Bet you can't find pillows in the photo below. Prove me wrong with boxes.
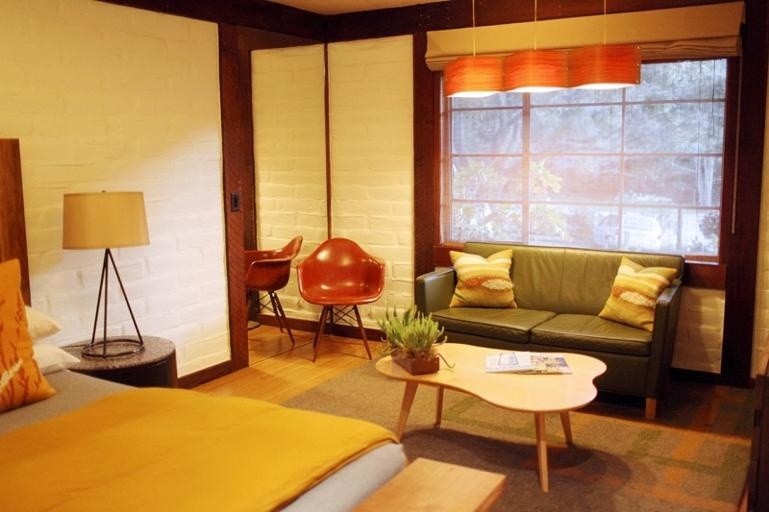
[448,248,517,310]
[24,306,60,341]
[31,342,80,375]
[601,254,676,333]
[0,257,55,414]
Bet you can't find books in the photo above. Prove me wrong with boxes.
[487,349,570,375]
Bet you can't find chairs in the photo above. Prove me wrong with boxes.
[244,235,302,344]
[298,238,384,361]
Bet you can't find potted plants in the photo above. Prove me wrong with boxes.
[377,305,456,374]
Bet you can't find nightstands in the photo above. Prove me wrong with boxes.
[55,335,177,388]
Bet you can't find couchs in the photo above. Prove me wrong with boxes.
[415,241,685,421]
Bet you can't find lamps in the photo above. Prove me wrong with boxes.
[443,56,501,100]
[504,48,567,95]
[570,45,642,92]
[57,189,149,359]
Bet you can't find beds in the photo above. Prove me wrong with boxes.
[0,138,407,512]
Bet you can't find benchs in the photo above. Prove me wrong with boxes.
[353,455,509,512]
